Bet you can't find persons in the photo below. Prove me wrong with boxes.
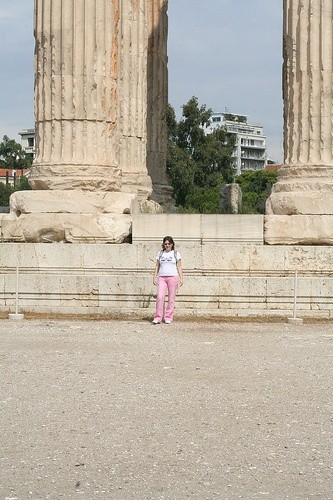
[152,236,184,325]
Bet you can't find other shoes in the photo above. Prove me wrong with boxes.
[164,320,171,324]
[151,318,162,324]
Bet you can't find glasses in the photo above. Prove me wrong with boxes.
[163,243,172,245]
